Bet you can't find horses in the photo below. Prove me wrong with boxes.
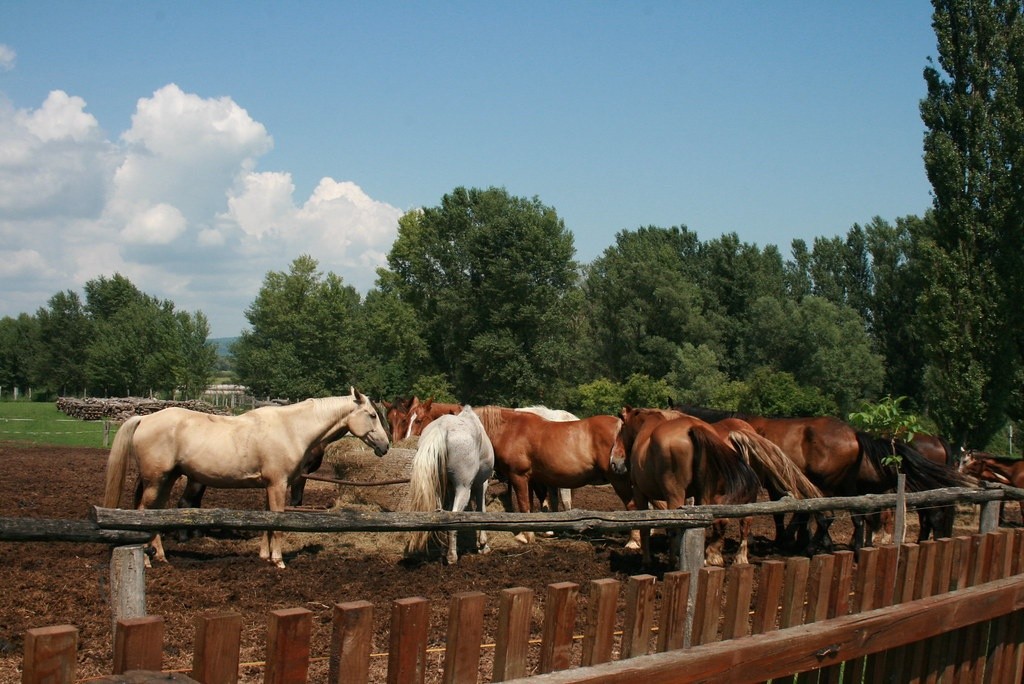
[472,406,643,550]
[609,406,765,568]
[404,404,494,566]
[380,395,413,447]
[513,404,581,536]
[402,395,463,439]
[739,415,988,563]
[958,445,1024,525]
[644,407,832,565]
[103,384,389,570]
[844,425,957,564]
